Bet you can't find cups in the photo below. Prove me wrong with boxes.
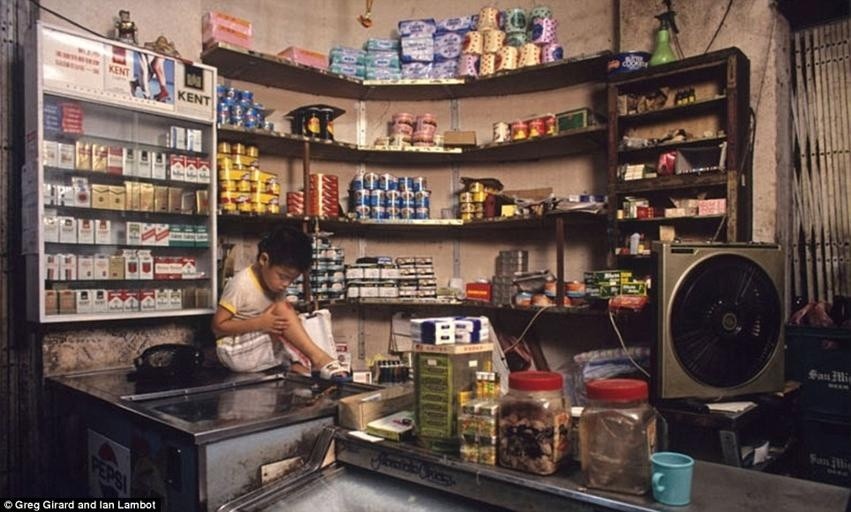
[648,451,696,507]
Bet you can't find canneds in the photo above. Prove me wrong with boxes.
[352,172,431,218]
[218,141,280,215]
[457,181,486,218]
[514,281,584,307]
[373,113,439,148]
[284,234,347,299]
[216,84,266,129]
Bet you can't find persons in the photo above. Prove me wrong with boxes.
[130,51,170,103]
[114,9,138,45]
[211,229,352,383]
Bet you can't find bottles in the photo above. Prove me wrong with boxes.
[498,370,573,478]
[578,378,657,497]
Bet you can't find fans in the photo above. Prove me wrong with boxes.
[657,243,787,399]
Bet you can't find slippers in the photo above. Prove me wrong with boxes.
[310,359,353,382]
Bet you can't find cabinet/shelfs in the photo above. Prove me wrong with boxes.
[24,20,218,324]
[465,50,660,322]
[201,42,363,313]
[608,46,750,262]
[364,76,465,307]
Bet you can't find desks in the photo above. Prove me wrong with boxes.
[610,371,813,479]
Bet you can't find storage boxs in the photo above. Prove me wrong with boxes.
[787,326,851,420]
[795,411,851,488]
